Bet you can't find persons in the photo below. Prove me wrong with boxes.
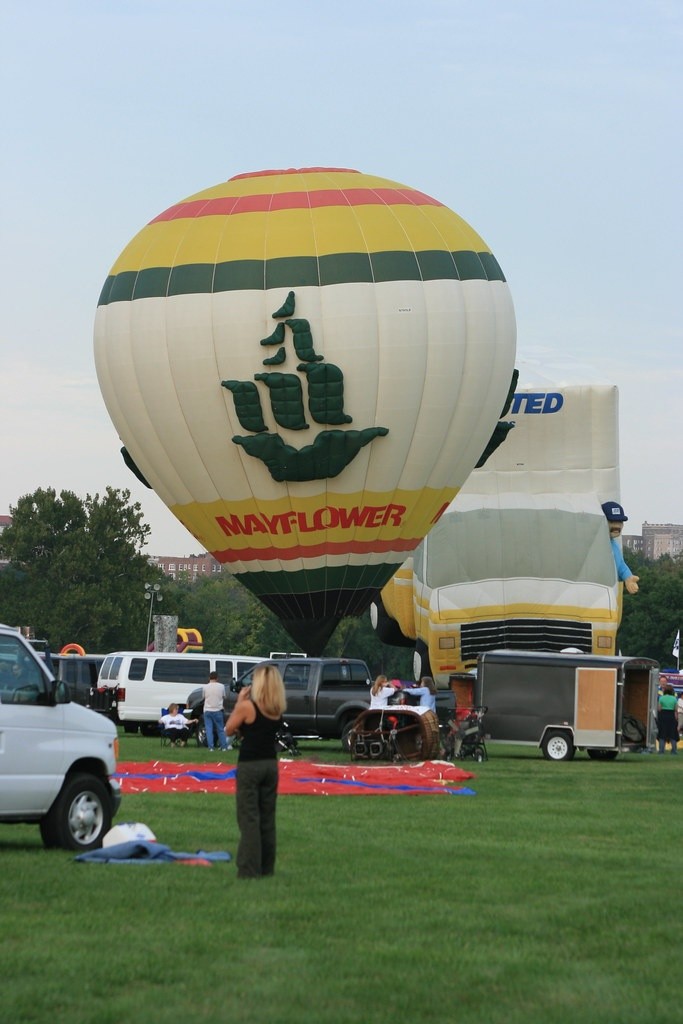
[226,665,287,880]
[601,502,639,594]
[657,675,683,755]
[186,672,228,752]
[402,677,437,713]
[388,679,405,705]
[158,703,199,747]
[370,675,397,708]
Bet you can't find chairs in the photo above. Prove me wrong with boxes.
[443,705,488,763]
[160,708,184,747]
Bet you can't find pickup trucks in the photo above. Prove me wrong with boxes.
[195,657,456,752]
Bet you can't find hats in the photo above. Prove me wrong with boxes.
[602,502,628,521]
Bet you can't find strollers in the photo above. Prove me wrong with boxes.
[441,706,487,761]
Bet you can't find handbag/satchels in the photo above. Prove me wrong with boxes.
[622,714,646,741]
[103,823,158,850]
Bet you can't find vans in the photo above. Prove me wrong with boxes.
[89,649,304,736]
[0,624,119,850]
[0,652,108,701]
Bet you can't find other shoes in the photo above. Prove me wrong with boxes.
[657,751,664,754]
[671,750,678,754]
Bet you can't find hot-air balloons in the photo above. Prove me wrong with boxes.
[95,167,516,661]
